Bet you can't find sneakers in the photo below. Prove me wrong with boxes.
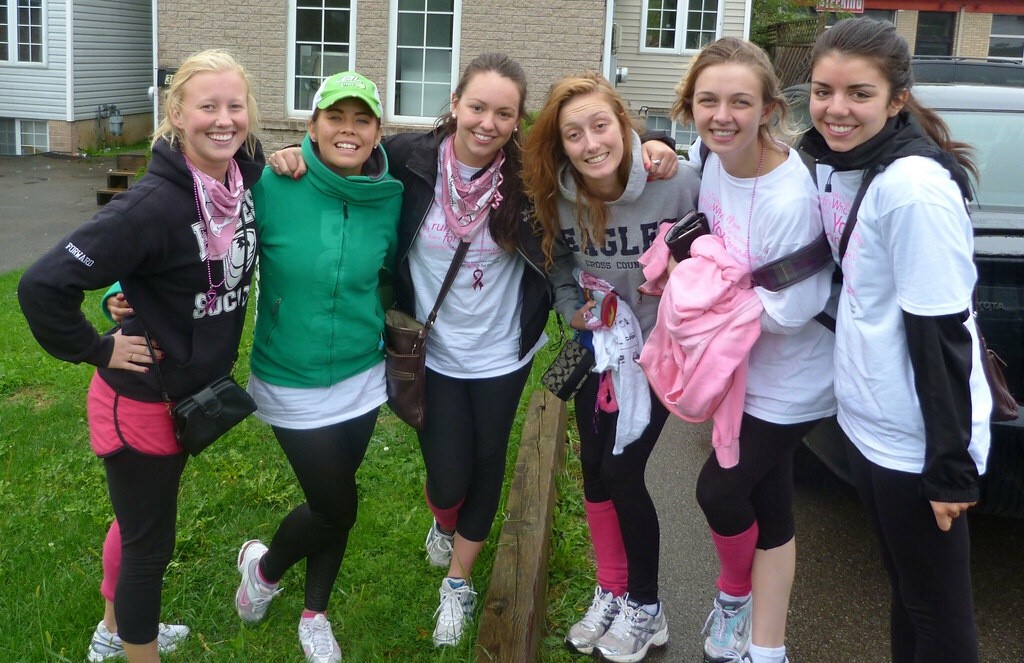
[235,539,279,622]
[299,610,343,663]
[565,587,669,663]
[431,578,477,647]
[87,618,191,663]
[702,591,789,663]
[427,519,454,568]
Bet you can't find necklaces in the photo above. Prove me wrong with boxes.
[442,139,502,289]
[718,148,766,282]
[182,152,234,313]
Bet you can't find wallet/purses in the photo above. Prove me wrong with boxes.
[540,339,597,402]
[664,210,711,263]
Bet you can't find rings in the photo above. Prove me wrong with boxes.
[273,164,279,167]
[651,159,661,165]
[129,352,133,361]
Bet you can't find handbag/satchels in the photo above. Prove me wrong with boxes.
[385,308,430,431]
[979,336,1018,422]
[170,376,259,457]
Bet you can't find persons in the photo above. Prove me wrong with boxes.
[669,36,844,663]
[800,17,994,663]
[18,49,265,663]
[102,72,405,663]
[520,70,703,663]
[268,52,679,645]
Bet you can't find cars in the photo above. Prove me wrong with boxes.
[910,57,1023,431]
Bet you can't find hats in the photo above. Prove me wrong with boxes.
[312,72,383,119]
